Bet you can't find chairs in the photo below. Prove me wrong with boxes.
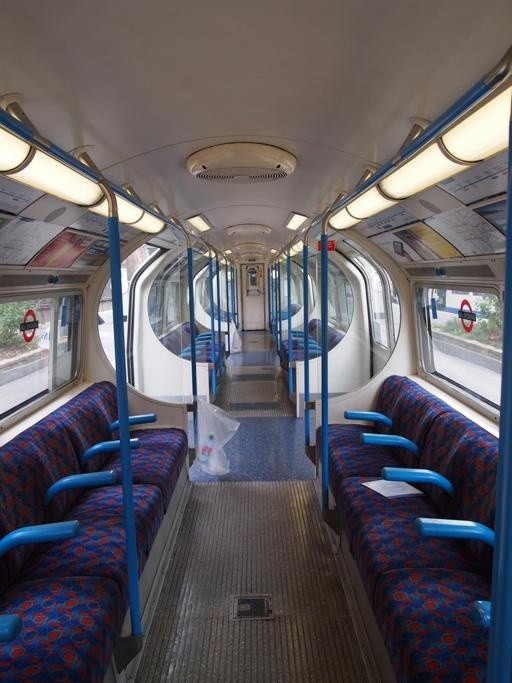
[268,304,303,338]
[279,318,345,406]
[315,374,501,680]
[1,380,188,683]
[157,319,230,402]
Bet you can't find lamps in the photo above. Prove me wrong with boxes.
[328,84,512,231]
[0,126,165,233]
